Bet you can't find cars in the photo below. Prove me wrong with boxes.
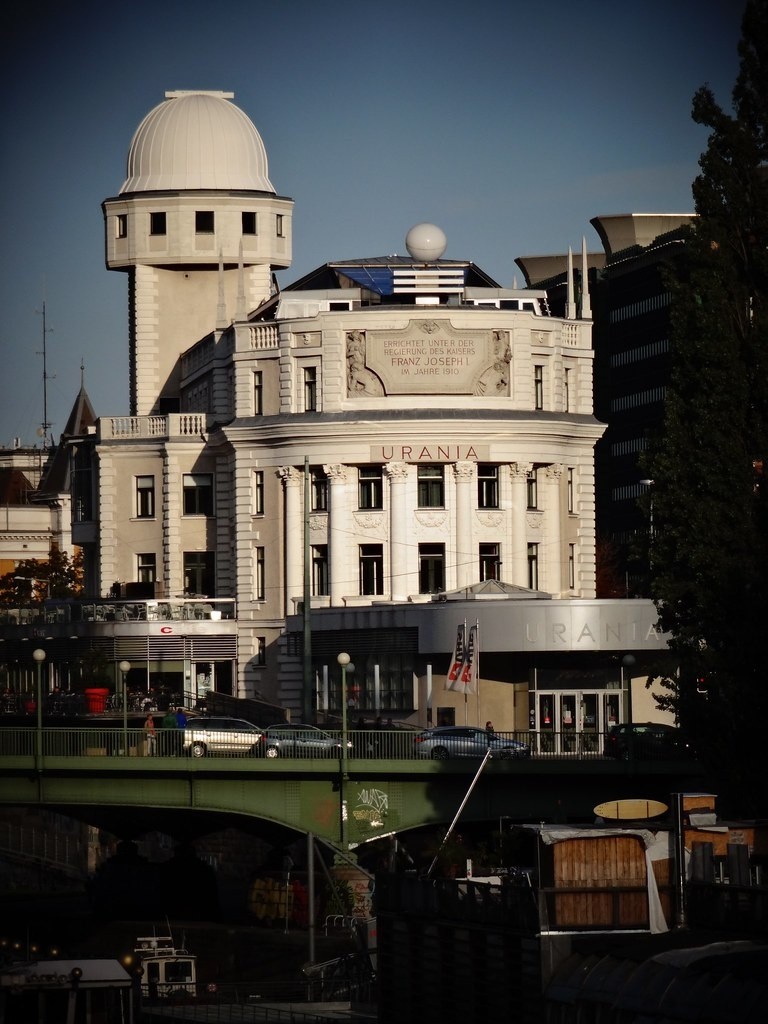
[255,722,355,760]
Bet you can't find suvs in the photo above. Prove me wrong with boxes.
[603,722,693,764]
[178,717,264,758]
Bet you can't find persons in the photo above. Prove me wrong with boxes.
[486,721,494,733]
[143,714,158,757]
[163,708,187,728]
[356,717,395,731]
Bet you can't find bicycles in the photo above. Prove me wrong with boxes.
[0,690,156,713]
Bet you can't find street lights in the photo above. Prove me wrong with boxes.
[118,661,131,757]
[333,651,360,867]
[33,648,46,802]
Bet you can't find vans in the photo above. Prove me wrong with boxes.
[411,725,532,762]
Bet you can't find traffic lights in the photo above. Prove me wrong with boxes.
[696,674,709,693]
